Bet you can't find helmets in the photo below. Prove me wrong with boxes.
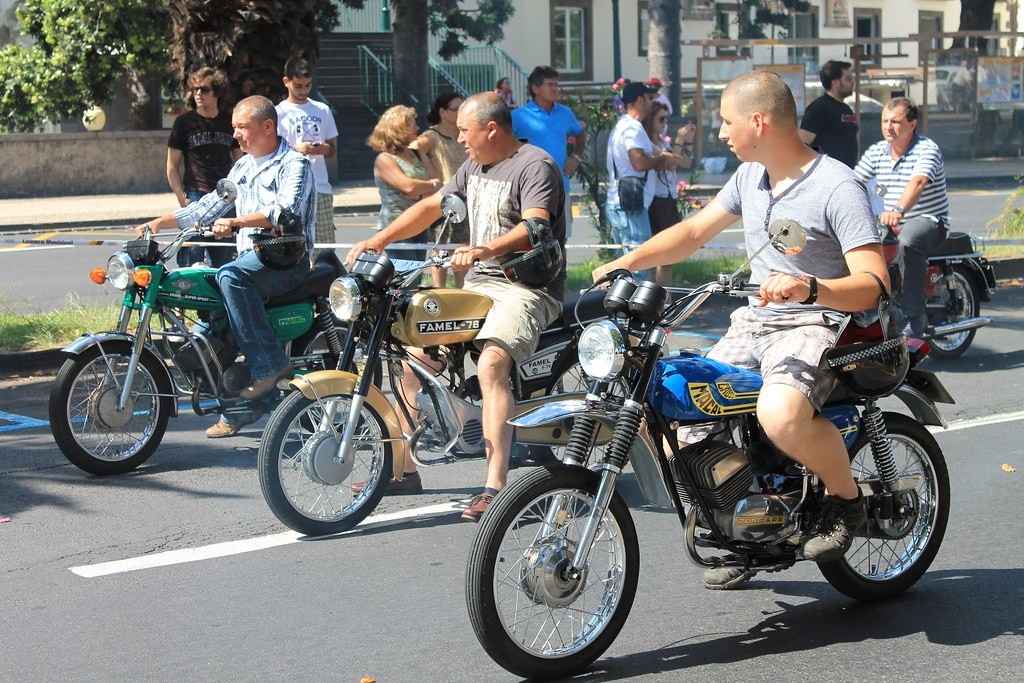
[248,209,306,270]
[501,239,563,289]
[825,293,910,398]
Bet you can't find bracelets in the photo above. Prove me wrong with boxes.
[431,179,438,193]
[684,143,694,145]
[675,143,683,148]
[570,153,582,164]
[799,275,818,305]
[683,147,695,160]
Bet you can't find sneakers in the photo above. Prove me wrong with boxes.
[239,360,292,400]
[461,493,497,521]
[206,411,264,437]
[352,471,422,496]
[803,485,867,562]
[704,566,792,590]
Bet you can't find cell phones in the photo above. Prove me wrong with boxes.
[312,142,320,146]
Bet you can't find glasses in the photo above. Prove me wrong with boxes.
[654,117,665,123]
[191,86,213,95]
[448,108,458,112]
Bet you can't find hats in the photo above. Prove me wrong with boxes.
[621,82,657,102]
[612,79,624,90]
[646,78,661,86]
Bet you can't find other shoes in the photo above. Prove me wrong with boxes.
[902,315,929,337]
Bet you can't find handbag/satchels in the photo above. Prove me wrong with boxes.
[618,176,644,211]
[647,193,679,236]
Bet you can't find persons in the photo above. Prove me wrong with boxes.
[167,68,244,333]
[606,77,696,318]
[494,77,518,111]
[365,105,440,291]
[952,60,975,109]
[273,59,339,267]
[591,68,891,590]
[410,92,471,290]
[135,95,317,439]
[798,60,859,169]
[511,66,585,245]
[346,91,567,522]
[854,96,950,336]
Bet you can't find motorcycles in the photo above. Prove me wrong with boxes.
[48,178,384,477]
[870,184,996,360]
[257,193,670,536]
[464,217,994,683]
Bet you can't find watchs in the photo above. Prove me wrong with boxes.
[892,206,906,218]
